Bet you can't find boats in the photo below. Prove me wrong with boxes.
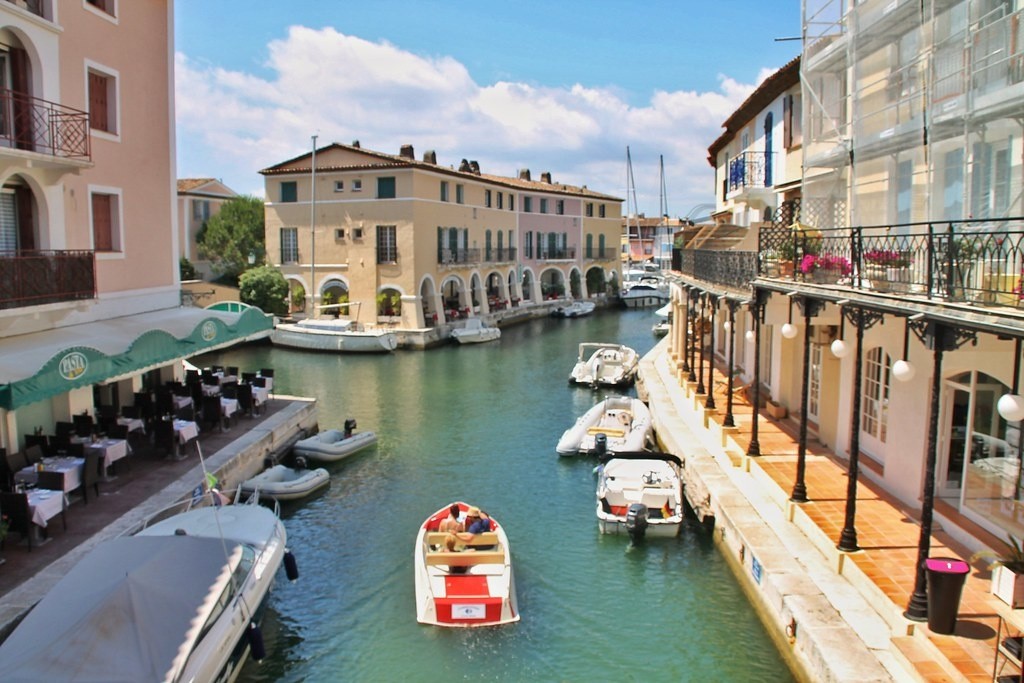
[652,320,670,337]
[568,342,641,388]
[594,453,685,543]
[292,418,379,462]
[555,394,653,456]
[0,483,300,682]
[554,301,595,317]
[239,465,329,502]
[414,501,519,628]
[450,319,501,345]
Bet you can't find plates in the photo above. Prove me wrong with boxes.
[42,456,76,469]
[26,488,51,506]
[70,437,115,448]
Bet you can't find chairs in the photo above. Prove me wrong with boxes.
[715,365,754,406]
[0,365,276,554]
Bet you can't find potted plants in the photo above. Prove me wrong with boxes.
[766,400,786,419]
[968,533,1024,609]
[756,238,798,279]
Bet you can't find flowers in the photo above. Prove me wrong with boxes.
[1011,267,1024,301]
[797,214,1008,278]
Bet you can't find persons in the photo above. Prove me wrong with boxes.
[438,504,495,575]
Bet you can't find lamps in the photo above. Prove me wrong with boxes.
[781,295,1023,422]
[695,290,756,343]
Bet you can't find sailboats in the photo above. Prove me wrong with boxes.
[617,145,671,307]
[269,134,398,353]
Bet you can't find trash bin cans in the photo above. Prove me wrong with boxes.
[921,556,971,636]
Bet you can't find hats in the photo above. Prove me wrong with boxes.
[466,506,479,517]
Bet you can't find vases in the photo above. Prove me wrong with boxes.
[812,264,913,295]
[940,262,970,302]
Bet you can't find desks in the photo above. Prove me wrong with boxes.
[16,488,70,543]
[14,455,88,505]
[115,417,146,444]
[250,386,270,418]
[200,383,221,397]
[220,397,242,430]
[920,556,970,635]
[213,372,237,386]
[70,436,133,477]
[171,420,200,455]
[256,375,274,400]
[149,393,194,420]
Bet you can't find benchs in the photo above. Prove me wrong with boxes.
[426,533,505,576]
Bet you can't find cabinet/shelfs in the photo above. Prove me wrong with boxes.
[991,609,1024,683]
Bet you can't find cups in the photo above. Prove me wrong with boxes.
[33,463,38,473]
[92,434,96,442]
[38,464,43,472]
